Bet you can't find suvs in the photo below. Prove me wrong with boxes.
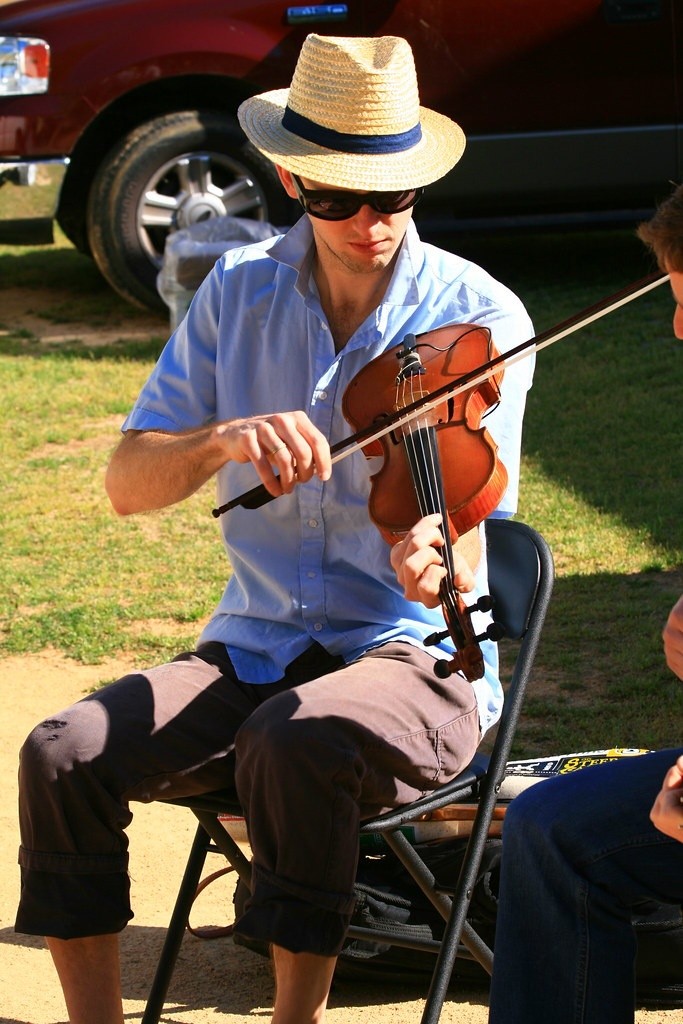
[0,0,683,320]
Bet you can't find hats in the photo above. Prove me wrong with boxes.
[236,32,467,193]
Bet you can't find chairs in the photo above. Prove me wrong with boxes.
[140,518,556,1024]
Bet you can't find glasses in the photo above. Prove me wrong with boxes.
[290,172,424,221]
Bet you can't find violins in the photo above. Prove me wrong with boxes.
[345,324,505,681]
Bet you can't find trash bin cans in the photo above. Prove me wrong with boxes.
[153,221,294,345]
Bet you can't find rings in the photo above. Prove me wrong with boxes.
[270,443,286,456]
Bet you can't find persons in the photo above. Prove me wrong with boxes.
[11,33,539,1024]
[488,185,683,1024]
